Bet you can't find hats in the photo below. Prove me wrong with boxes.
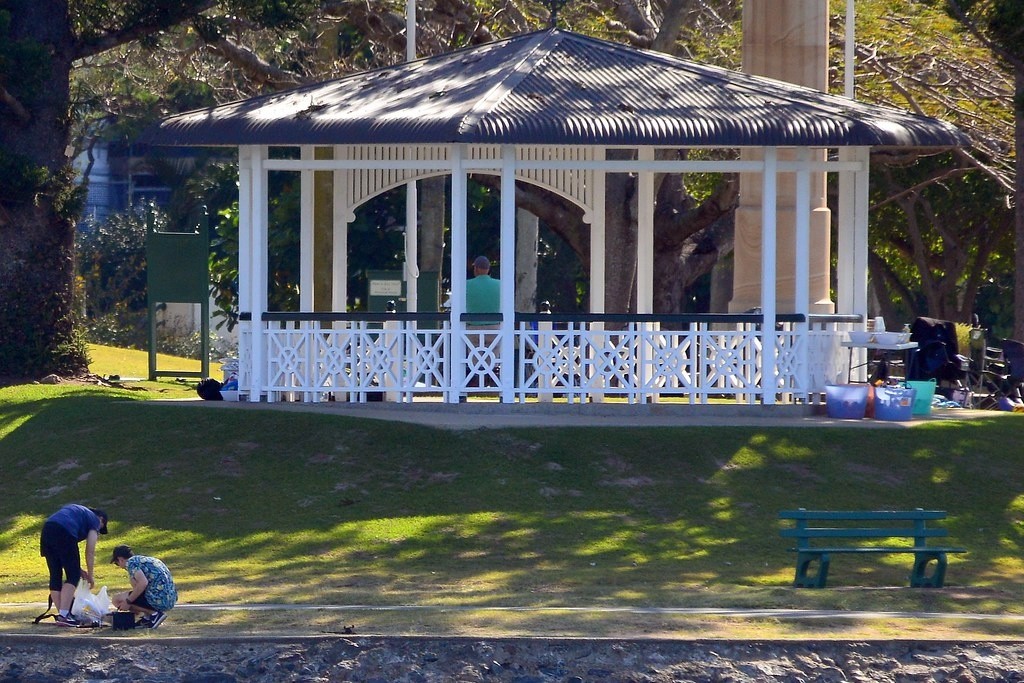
[94,508,108,534]
[471,256,490,269]
[109,545,132,564]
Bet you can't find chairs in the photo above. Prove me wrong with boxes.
[966,339,1024,410]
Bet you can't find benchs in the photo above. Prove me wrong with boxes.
[776,508,968,592]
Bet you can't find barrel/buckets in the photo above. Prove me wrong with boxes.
[822,377,871,419]
[874,383,916,421]
[897,377,936,415]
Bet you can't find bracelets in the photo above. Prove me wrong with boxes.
[126,599,135,604]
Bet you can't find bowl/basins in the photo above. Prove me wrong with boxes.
[873,332,912,345]
[848,331,874,343]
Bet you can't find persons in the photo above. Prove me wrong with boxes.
[466,255,500,387]
[197,373,288,403]
[442,283,451,313]
[112,544,178,629]
[531,301,557,348]
[40,502,108,626]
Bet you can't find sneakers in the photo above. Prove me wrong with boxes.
[56,612,79,626]
[134,616,152,628]
[147,609,166,628]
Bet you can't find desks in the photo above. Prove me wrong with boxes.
[840,340,919,385]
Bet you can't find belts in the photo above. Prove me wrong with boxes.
[466,322,500,326]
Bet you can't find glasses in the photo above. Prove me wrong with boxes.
[114,559,120,566]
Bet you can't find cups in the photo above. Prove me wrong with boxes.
[874,316,886,333]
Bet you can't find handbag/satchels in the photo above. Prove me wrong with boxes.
[70,577,110,627]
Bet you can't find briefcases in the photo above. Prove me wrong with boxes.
[111,607,136,630]
